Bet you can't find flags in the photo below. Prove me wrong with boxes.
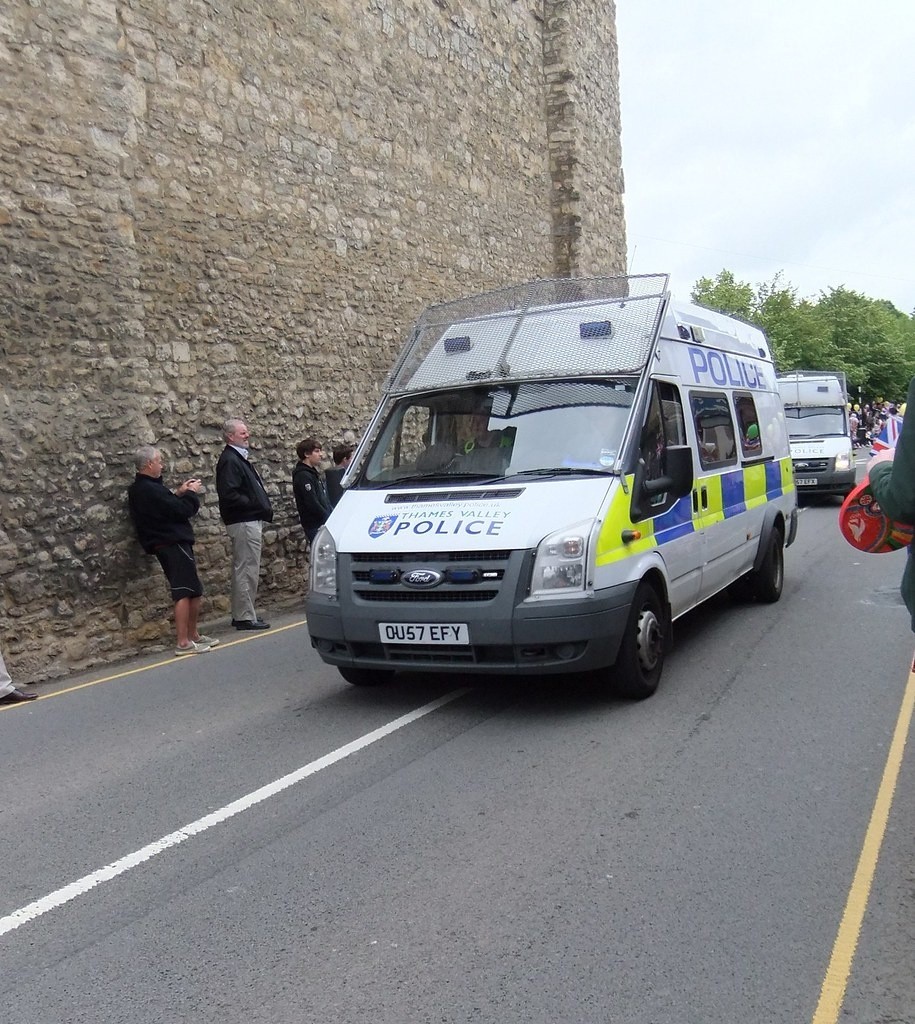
[870,417,904,457]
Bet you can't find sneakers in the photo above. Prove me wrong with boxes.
[195,635,219,647]
[174,640,210,655]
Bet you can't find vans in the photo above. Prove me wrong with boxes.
[775,369,867,503]
[299,272,801,700]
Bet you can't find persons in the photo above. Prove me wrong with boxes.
[458,412,514,456]
[216,420,273,629]
[849,400,902,449]
[0,652,38,703]
[325,444,352,507]
[866,376,915,673]
[291,438,335,544]
[127,446,219,656]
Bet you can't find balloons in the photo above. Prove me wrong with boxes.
[748,424,758,438]
[854,404,859,410]
[847,403,852,409]
[884,401,889,406]
[900,403,907,416]
[876,397,883,403]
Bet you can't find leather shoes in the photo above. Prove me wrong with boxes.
[232,617,263,627]
[236,619,270,630]
[0,688,38,705]
